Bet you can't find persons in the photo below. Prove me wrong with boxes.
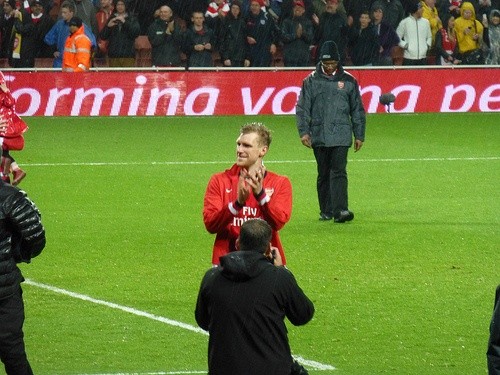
[0,0,500,72]
[202,123,293,269]
[0,71,29,186]
[296,40,366,223]
[195,218,315,374]
[0,181,46,375]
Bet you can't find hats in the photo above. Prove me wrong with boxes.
[30,0,42,7]
[293,0,304,7]
[319,41,340,60]
[67,16,82,27]
[253,0,264,6]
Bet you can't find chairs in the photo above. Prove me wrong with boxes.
[0,37,440,69]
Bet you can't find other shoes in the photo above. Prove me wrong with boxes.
[320,212,332,219]
[334,210,354,222]
